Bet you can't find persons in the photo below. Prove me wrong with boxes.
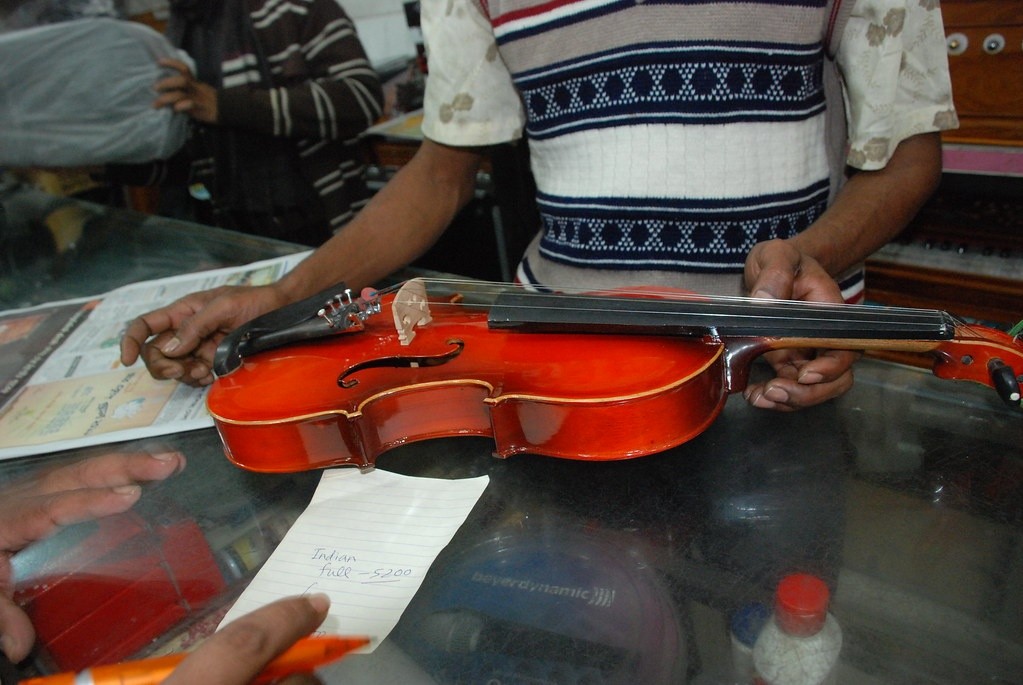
[0,449,333,685]
[113,1,960,418]
[100,0,387,252]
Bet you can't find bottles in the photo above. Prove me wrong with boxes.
[729,602,774,678]
[751,572,844,683]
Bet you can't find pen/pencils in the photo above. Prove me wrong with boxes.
[14,633,378,685]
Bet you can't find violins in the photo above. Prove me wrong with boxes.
[206,278,1023,473]
